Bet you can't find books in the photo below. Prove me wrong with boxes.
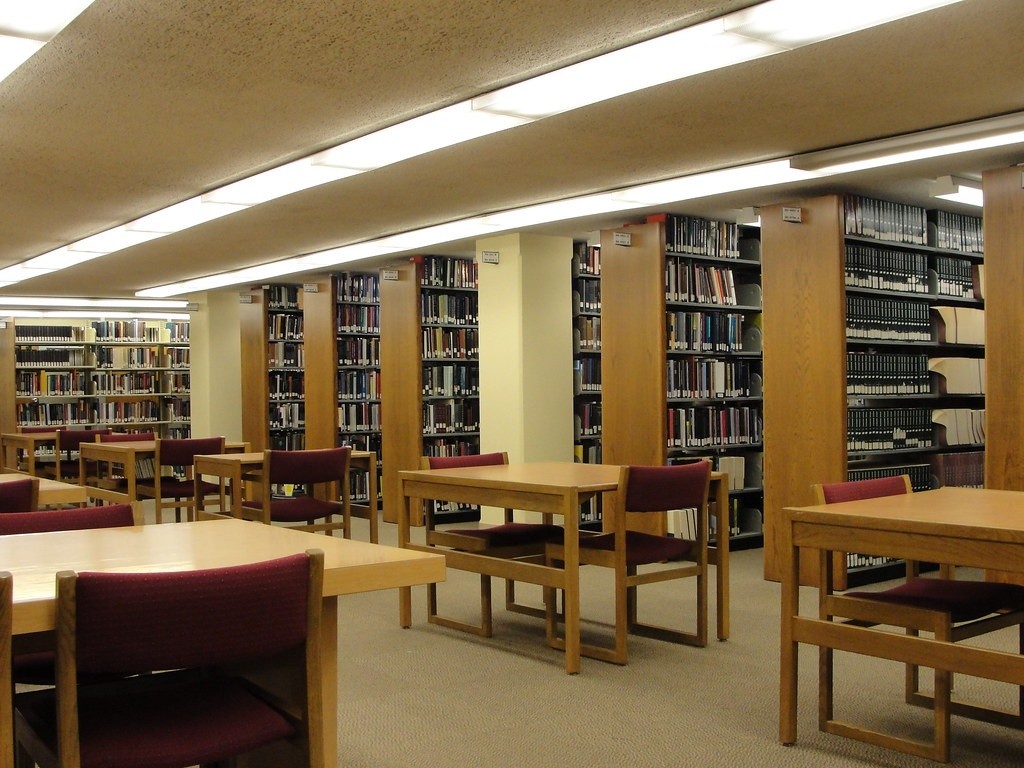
[6,192,988,578]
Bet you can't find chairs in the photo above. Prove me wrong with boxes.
[0,571,15,768]
[0,425,1024,767]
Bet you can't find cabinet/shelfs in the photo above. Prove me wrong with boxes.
[239,283,309,504]
[303,271,384,510]
[758,189,986,591]
[378,256,482,527]
[599,213,763,564]
[1,309,192,482]
[477,232,602,531]
